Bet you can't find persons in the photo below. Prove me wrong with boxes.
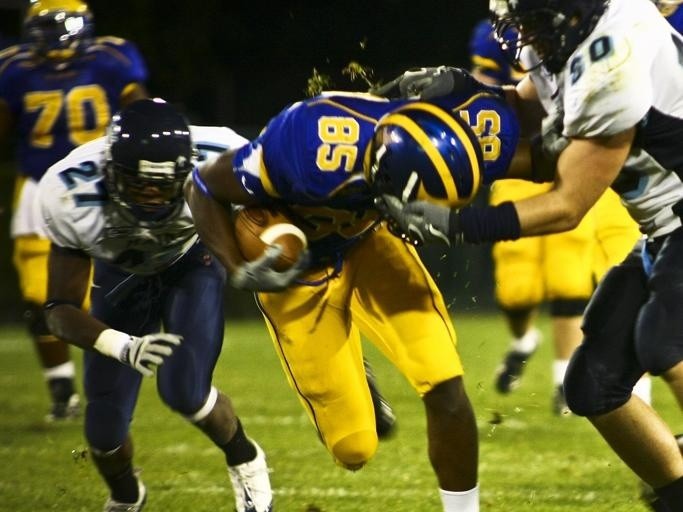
[0,0,146,421]
[469,14,606,414]
[182,91,559,511]
[375,1,683,512]
[594,0,683,407]
[32,97,273,512]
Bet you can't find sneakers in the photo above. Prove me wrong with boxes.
[45,393,79,426]
[103,479,146,512]
[226,438,273,512]
[496,332,541,391]
[552,386,574,417]
[363,358,396,439]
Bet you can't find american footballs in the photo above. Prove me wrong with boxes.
[233,208,308,273]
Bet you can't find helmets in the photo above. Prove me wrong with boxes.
[490,0,610,73]
[364,104,482,211]
[104,98,192,220]
[21,0,91,68]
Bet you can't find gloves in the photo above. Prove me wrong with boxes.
[532,126,574,186]
[372,67,466,102]
[95,327,181,377]
[230,248,298,294]
[374,195,448,246]
